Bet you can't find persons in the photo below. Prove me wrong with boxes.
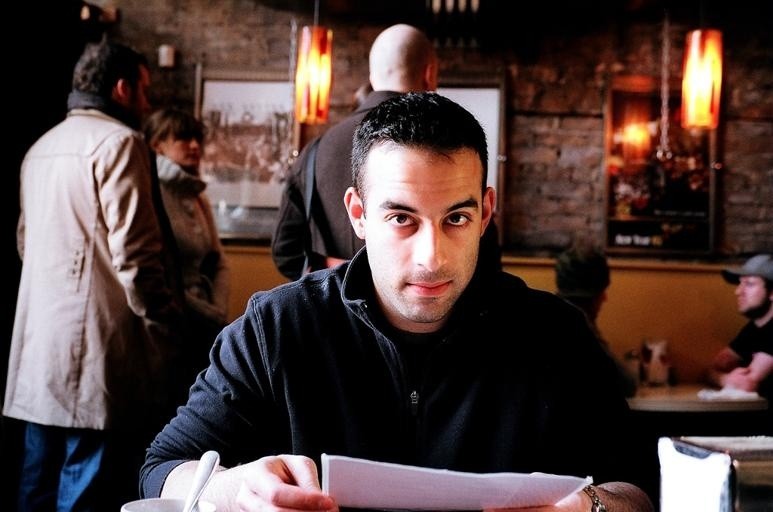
[0,33,190,511]
[137,92,658,510]
[270,22,502,280]
[549,224,638,400]
[704,252,772,402]
[141,106,228,419]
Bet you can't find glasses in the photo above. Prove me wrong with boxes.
[291,1,334,132]
[677,2,725,141]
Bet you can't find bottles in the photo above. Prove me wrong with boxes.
[720,252,773,286]
[554,246,611,298]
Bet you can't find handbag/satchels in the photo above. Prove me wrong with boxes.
[582,482,604,511]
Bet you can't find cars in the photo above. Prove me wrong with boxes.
[121,497,213,512]
[620,359,640,384]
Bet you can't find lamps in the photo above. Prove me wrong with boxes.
[653,433,739,512]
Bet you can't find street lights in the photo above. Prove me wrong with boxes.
[298,247,353,280]
